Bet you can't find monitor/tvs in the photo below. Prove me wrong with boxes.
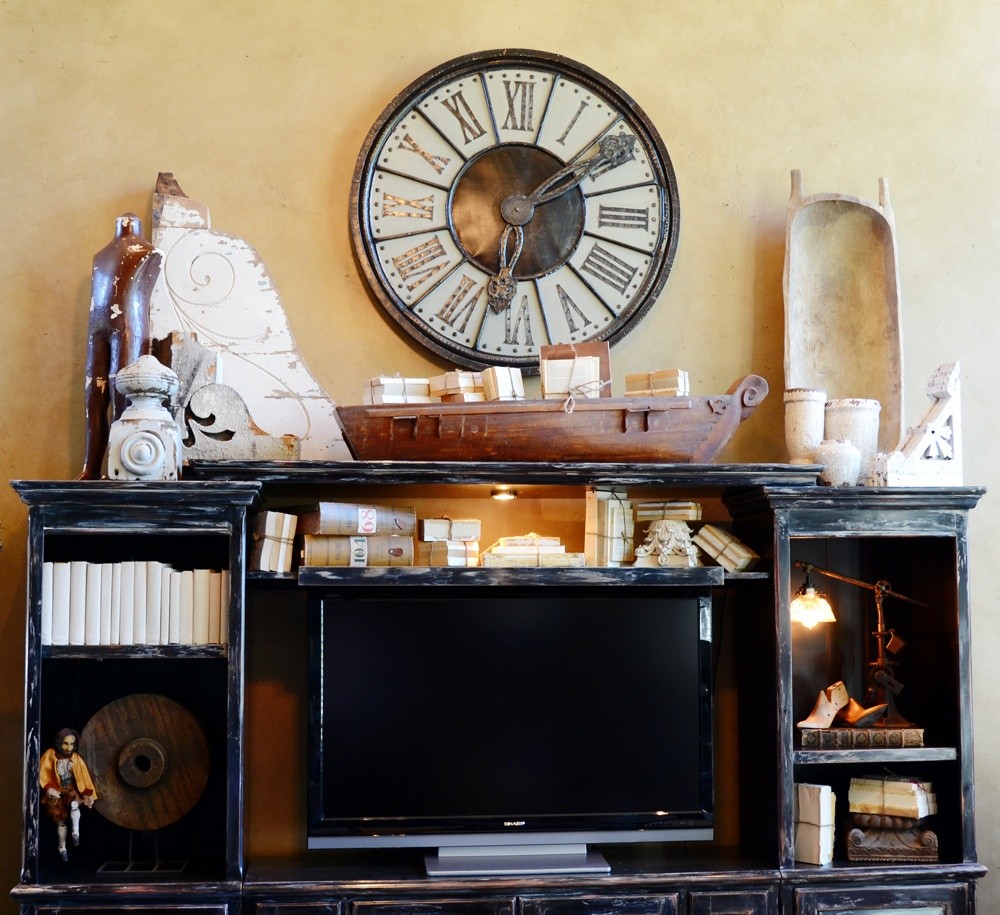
[304,587,715,875]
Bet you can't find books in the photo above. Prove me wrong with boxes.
[42,562,229,645]
[249,490,761,571]
[363,341,690,404]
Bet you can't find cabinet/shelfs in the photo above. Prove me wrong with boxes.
[8,459,988,915]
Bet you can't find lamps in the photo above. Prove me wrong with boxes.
[791,560,932,732]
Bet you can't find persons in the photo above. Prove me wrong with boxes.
[39,729,98,862]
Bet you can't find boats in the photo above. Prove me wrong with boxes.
[332,375,770,463]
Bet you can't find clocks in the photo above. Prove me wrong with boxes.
[347,48,682,373]
